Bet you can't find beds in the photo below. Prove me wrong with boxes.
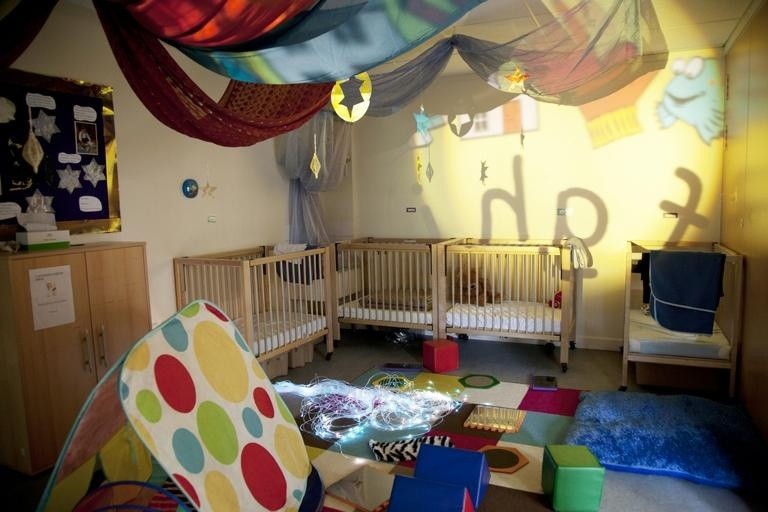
[334,235,437,354]
[174,244,333,363]
[619,242,744,397]
[436,236,582,375]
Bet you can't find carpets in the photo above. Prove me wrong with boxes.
[566,387,754,493]
[289,366,590,512]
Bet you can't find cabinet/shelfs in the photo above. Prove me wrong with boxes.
[0,239,152,475]
